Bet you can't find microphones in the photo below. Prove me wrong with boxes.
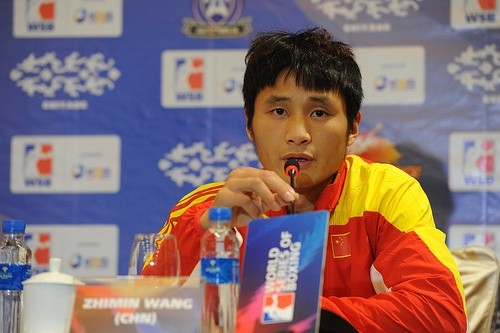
[283,158,300,214]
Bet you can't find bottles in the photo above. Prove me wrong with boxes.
[0,219,33,333]
[200,206,244,333]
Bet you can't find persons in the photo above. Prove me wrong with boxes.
[141,27,471,333]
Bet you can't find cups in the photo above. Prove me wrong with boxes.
[129,233,181,288]
[21,257,86,333]
